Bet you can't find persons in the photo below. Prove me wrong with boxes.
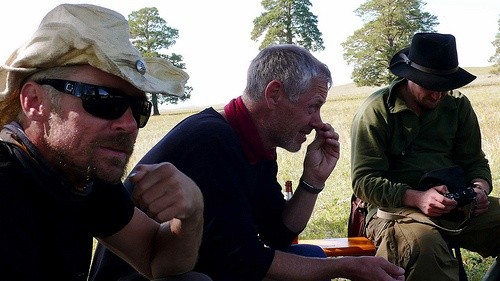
[88,45,404,281]
[350,33,500,281]
[0,3,204,281]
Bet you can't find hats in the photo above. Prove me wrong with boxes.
[0,3,189,136]
[387,32,476,90]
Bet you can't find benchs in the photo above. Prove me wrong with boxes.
[299,237,376,256]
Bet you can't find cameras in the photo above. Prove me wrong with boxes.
[443,187,477,207]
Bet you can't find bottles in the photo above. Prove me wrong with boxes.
[284,181,298,244]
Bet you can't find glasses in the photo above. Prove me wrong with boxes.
[34,78,151,129]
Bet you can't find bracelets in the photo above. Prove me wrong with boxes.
[476,185,488,197]
[300,177,325,194]
